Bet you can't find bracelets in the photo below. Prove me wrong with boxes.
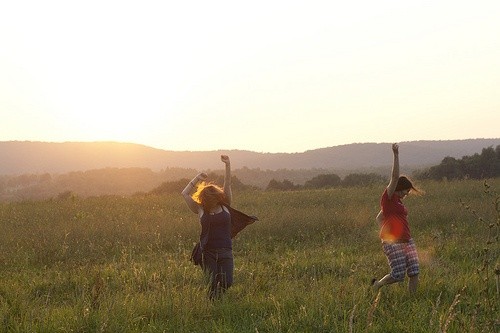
[190,182,195,187]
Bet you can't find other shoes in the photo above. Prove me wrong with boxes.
[368,279,377,292]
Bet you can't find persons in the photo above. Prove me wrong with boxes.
[367,144,423,301]
[181,154,258,302]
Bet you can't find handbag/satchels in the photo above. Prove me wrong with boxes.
[190,243,202,267]
